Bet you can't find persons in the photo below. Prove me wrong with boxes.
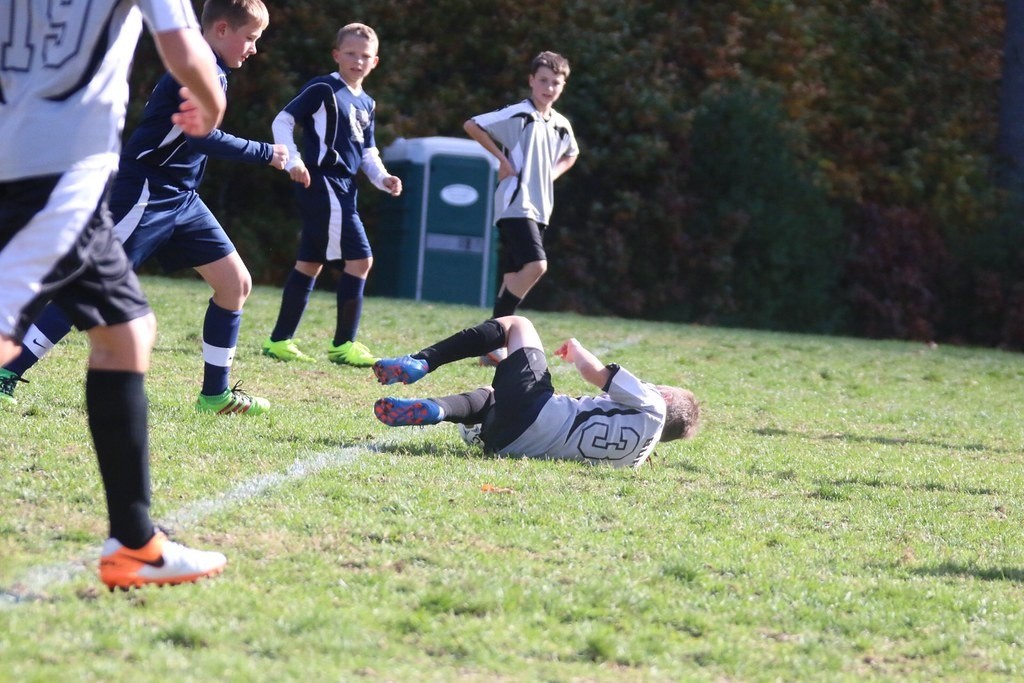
[0,1,272,592]
[372,315,699,469]
[262,23,402,368]
[462,51,580,367]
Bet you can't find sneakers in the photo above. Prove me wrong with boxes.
[194,379,271,417]
[374,396,444,427]
[373,353,429,386]
[97,528,228,594]
[0,366,29,408]
[328,338,382,369]
[260,335,317,364]
[479,348,507,366]
